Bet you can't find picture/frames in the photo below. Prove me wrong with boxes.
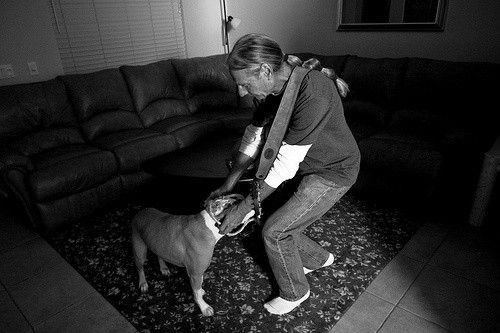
[336,0,450,33]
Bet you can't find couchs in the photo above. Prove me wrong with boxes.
[0,52,500,234]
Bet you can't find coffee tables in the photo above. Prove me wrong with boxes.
[163,136,255,191]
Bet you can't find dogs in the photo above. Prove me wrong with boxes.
[128,190,250,319]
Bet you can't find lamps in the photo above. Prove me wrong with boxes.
[223,0,242,53]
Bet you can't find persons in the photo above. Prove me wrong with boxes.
[205,32,362,315]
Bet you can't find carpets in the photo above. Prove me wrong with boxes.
[41,185,431,333]
